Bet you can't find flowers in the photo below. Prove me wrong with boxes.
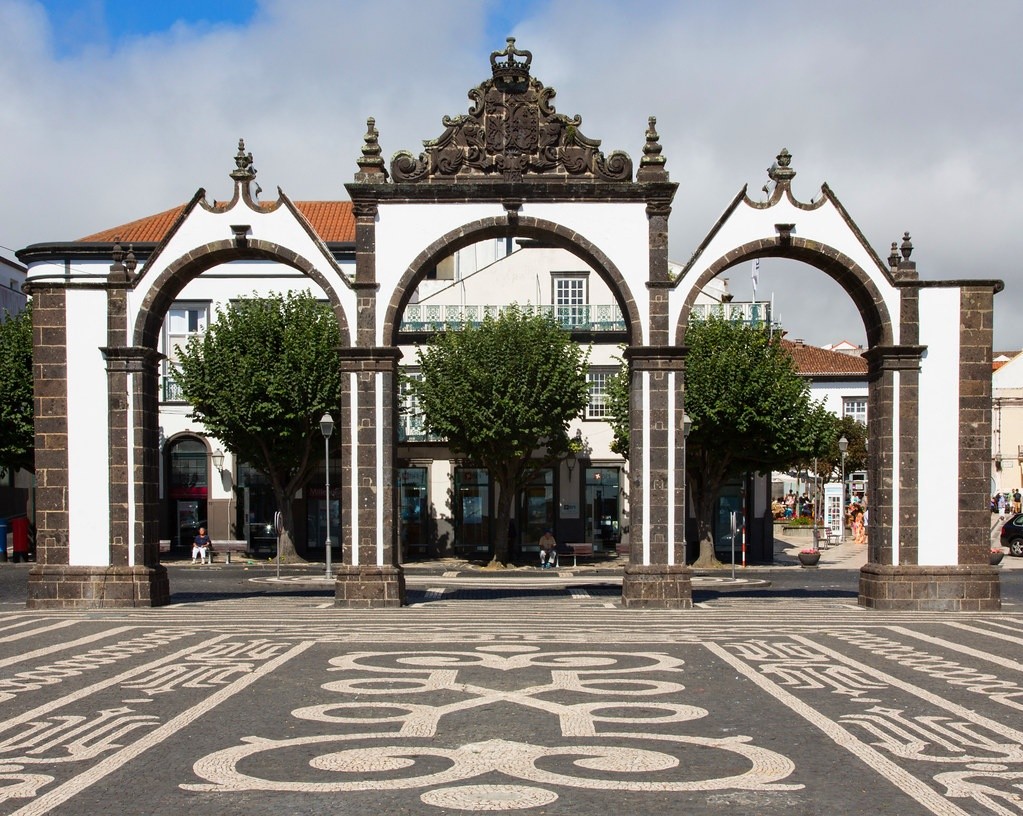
[801,548,818,553]
[776,510,824,526]
[991,549,1002,554]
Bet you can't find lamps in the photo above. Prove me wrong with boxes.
[565,451,577,483]
[212,448,225,481]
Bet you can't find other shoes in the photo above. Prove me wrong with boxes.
[201,559,206,565]
[191,559,197,564]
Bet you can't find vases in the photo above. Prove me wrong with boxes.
[783,526,824,537]
[798,552,821,565]
[774,521,789,534]
[990,553,1004,565]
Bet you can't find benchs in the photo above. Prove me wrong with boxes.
[159,540,171,563]
[206,539,248,564]
[545,543,592,567]
[615,543,629,557]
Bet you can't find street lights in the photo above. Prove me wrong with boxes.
[837,436,848,542]
[319,412,334,579]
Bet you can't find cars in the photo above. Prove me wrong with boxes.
[1000,513,1023,557]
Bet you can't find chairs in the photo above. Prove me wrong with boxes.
[825,530,840,546]
[813,531,828,550]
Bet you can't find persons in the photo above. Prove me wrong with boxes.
[191,528,211,565]
[848,491,869,544]
[772,489,815,519]
[538,531,556,568]
[991,489,1021,515]
